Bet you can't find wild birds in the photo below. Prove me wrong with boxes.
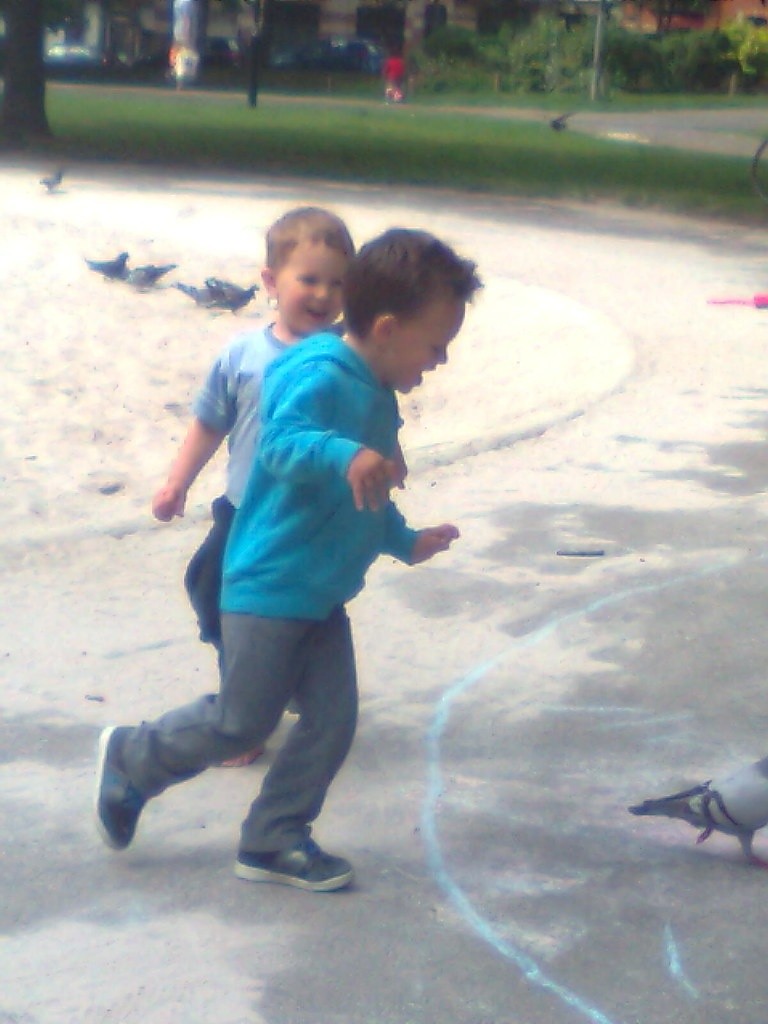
[38,170,64,193]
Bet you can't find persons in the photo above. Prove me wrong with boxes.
[150,208,355,767]
[382,44,405,101]
[95,229,482,889]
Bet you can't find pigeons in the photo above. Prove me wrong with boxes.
[84,250,260,314]
[627,754,768,870]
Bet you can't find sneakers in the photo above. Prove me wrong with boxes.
[94,724,144,851]
[235,836,353,891]
